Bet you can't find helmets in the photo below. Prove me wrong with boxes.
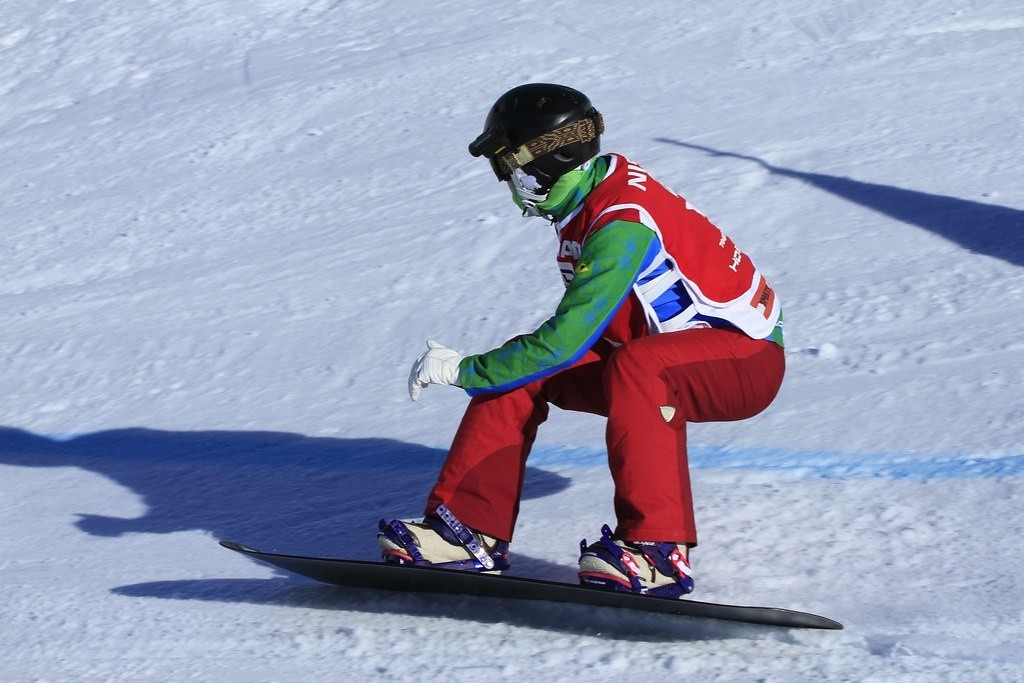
[468,82,605,183]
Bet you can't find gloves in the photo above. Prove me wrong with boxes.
[407,337,464,404]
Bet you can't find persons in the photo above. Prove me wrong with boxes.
[376,83,784,598]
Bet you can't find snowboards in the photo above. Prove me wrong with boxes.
[219,540,844,629]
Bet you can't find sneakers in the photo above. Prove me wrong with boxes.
[578,537,695,597]
[374,504,507,574]
